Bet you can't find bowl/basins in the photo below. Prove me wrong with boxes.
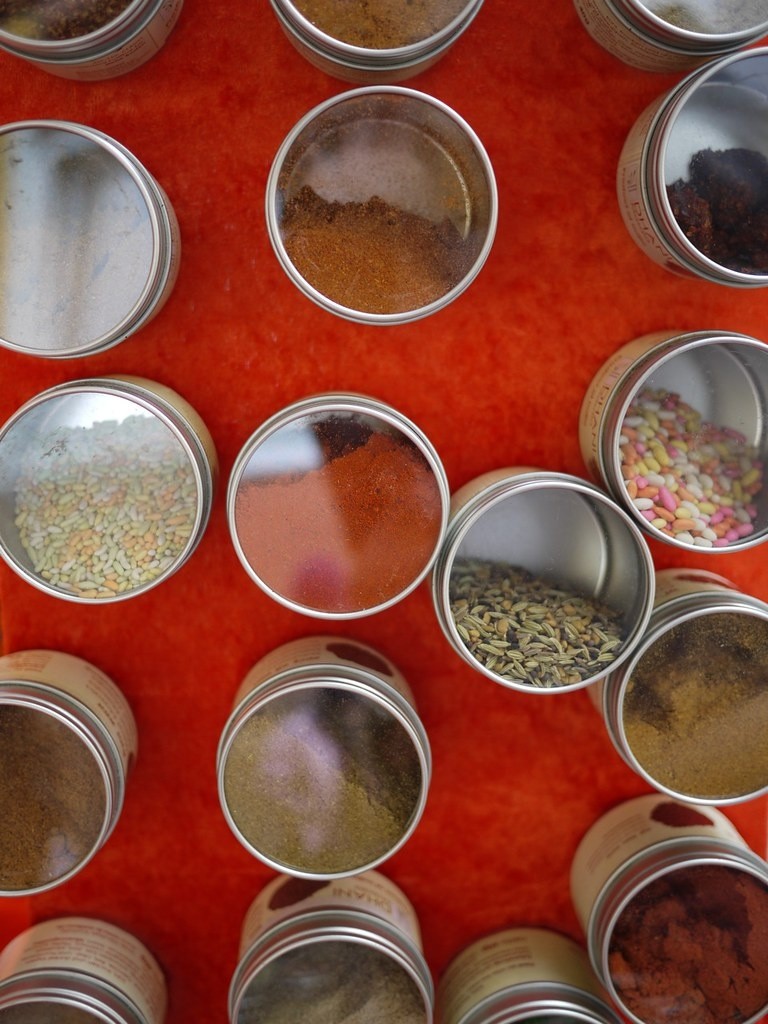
[0,0,187,85]
[435,923,624,1024]
[584,569,768,806]
[577,326,768,556]
[226,390,451,622]
[226,870,434,1024]
[570,0,768,75]
[0,649,138,899]
[0,373,220,605]
[615,45,768,298]
[0,914,170,1024]
[565,792,768,1024]
[430,464,659,696]
[268,0,487,86]
[262,84,503,328]
[214,633,434,883]
[0,118,182,360]
